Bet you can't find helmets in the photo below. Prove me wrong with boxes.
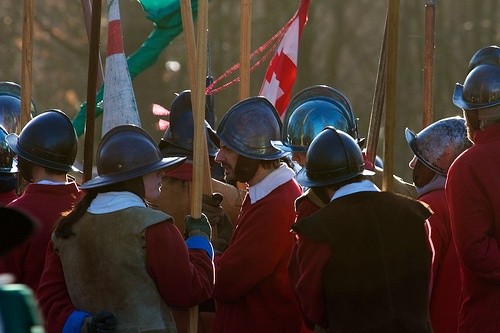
[451,45,500,110]
[360,147,384,173]
[0,207,37,247]
[404,119,473,177]
[79,124,187,190]
[0,82,37,150]
[5,109,78,172]
[204,96,292,160]
[158,89,221,158]
[270,85,366,152]
[295,126,375,186]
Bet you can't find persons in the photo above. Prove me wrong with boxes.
[205,96,313,333]
[146,113,249,333]
[36,125,217,333]
[0,206,47,333]
[289,125,436,333]
[0,109,88,296]
[403,114,475,333]
[0,93,31,134]
[443,63,500,333]
[271,94,423,236]
[0,123,25,205]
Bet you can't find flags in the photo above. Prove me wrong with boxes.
[257,0,313,129]
[101,0,140,144]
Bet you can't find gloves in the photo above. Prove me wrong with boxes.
[87,311,117,333]
[184,212,212,239]
[201,192,225,225]
